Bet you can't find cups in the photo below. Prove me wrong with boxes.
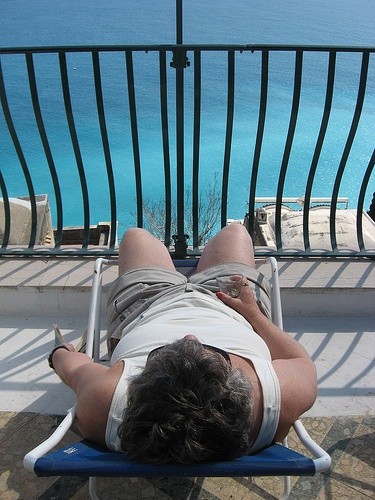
[216,269,244,299]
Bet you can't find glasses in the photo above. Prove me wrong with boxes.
[146,343,232,372]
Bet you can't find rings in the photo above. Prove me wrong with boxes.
[243,283,248,286]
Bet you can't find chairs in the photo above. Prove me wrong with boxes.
[20,256,333,500]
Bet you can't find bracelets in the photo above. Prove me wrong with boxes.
[48,345,70,369]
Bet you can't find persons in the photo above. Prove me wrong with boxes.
[48,220,317,467]
